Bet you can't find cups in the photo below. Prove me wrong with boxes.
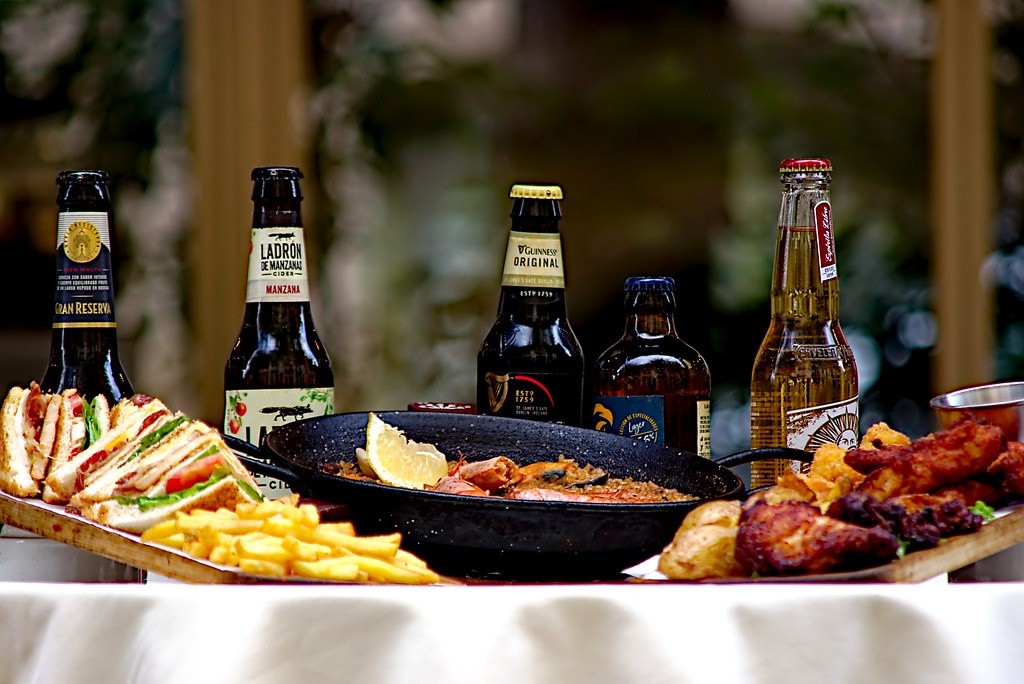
[930,381,1022,582]
[0,523,144,584]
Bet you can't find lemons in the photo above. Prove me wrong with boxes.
[367,412,448,490]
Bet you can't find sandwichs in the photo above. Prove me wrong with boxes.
[0,382,271,535]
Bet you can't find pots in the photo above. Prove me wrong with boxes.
[220,410,814,586]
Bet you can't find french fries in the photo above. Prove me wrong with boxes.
[137,489,449,587]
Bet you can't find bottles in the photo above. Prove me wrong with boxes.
[751,160,859,498]
[594,274,712,463]
[38,171,135,403]
[220,166,334,448]
[474,184,584,432]
